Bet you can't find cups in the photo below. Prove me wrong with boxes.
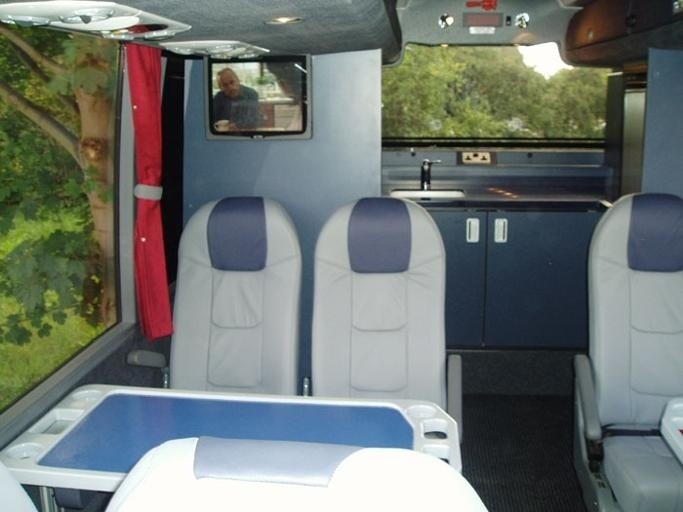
[213,119,231,132]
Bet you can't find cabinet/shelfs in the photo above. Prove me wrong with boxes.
[427,211,604,350]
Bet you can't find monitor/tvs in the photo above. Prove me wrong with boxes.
[204,53,313,142]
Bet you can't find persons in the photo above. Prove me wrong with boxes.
[212,66,259,131]
[266,60,303,132]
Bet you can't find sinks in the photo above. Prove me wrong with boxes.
[390,188,466,199]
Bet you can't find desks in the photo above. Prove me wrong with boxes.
[0,383,462,511]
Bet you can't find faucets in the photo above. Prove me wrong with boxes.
[420,159,443,189]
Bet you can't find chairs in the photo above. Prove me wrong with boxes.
[572,192,682,511]
